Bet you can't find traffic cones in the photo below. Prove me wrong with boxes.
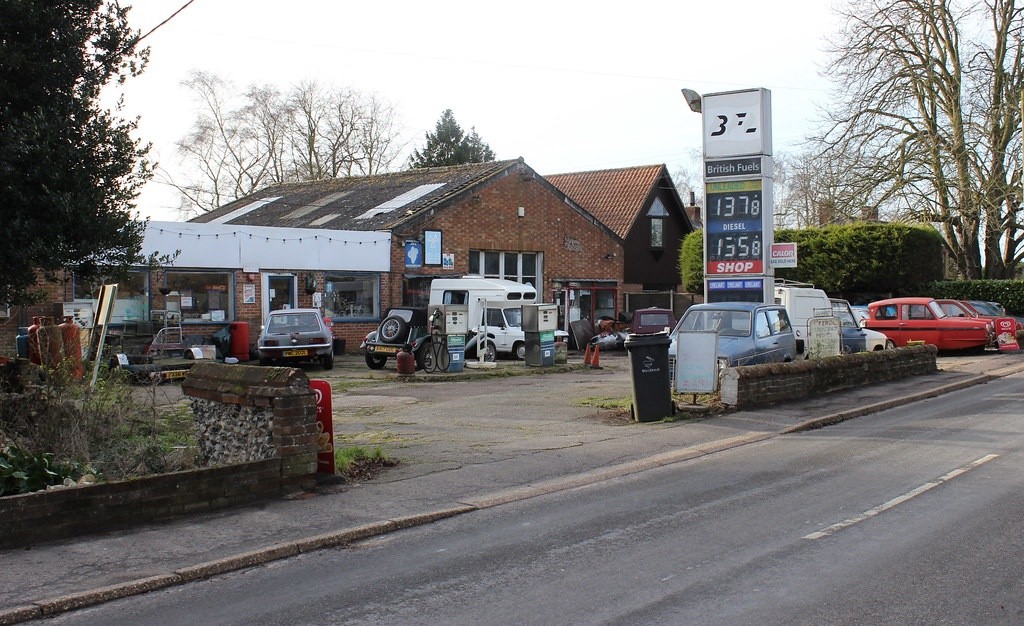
[589,345,604,370]
[583,342,592,365]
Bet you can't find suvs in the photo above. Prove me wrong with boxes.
[361,306,429,369]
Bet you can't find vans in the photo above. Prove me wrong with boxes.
[772,275,834,360]
[427,276,536,363]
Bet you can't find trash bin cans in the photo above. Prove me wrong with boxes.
[230,322,250,361]
[624,331,675,422]
[554,330,569,364]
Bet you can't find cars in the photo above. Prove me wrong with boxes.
[631,307,676,338]
[257,308,335,369]
[665,301,798,389]
[830,294,1024,354]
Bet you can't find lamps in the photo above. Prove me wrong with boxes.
[602,253,616,259]
[473,195,480,202]
